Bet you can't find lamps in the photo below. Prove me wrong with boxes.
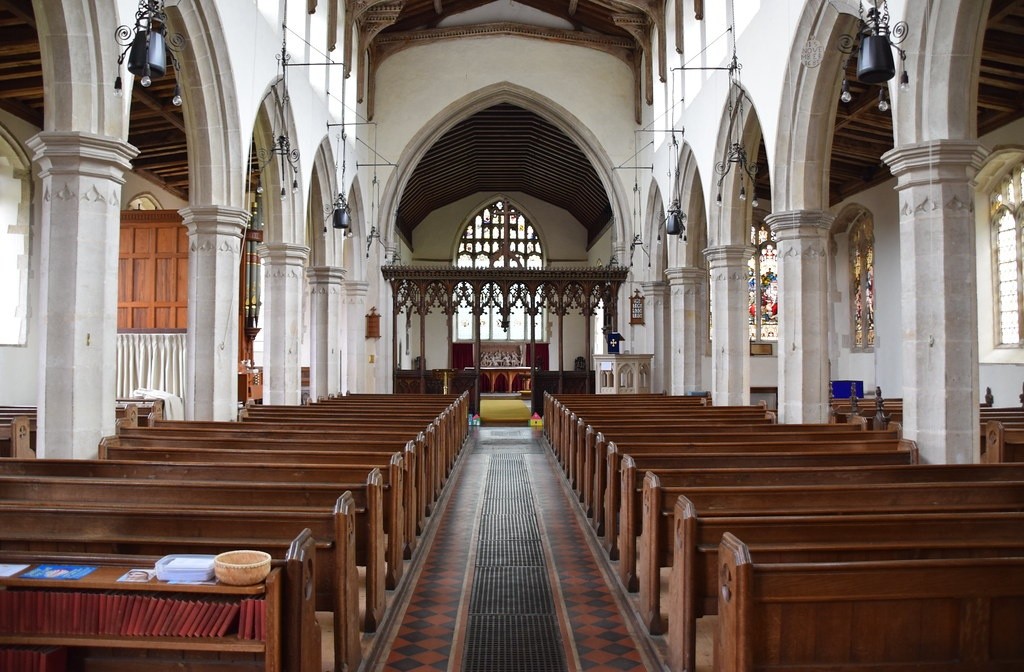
[113,1,187,106]
[646,130,689,249]
[624,181,655,277]
[714,48,762,207]
[365,123,402,264]
[836,1,910,112]
[322,133,354,242]
[251,25,300,201]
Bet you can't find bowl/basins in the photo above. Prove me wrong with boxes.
[214,550,272,586]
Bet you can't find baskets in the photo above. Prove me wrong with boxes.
[214,550,272,585]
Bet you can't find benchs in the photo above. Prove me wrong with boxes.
[0,385,472,672]
[540,379,1024,671]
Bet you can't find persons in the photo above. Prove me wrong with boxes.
[126,571,148,581]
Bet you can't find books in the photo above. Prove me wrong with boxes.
[0,647,67,672]
[0,591,266,643]
